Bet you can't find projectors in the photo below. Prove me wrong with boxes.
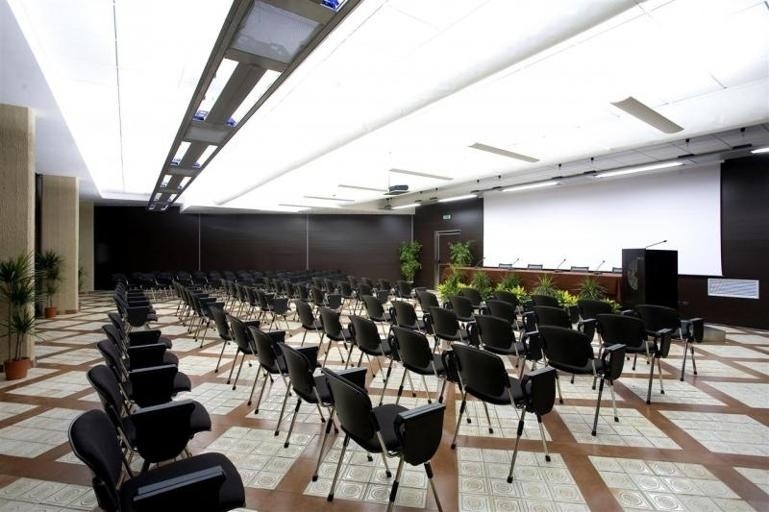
[389,185,408,192]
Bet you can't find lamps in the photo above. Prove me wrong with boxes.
[439,194,477,205]
[502,180,559,195]
[388,201,422,212]
[751,148,769,154]
[593,160,683,180]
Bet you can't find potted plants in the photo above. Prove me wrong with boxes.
[0,245,49,381]
[32,249,67,319]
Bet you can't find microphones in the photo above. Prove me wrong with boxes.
[509,258,519,270]
[554,259,566,272]
[645,240,667,248]
[593,260,605,275]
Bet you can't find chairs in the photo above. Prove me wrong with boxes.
[275,342,368,448]
[394,325,473,423]
[171,274,705,404]
[69,410,246,511]
[87,364,211,463]
[312,367,447,512]
[96,281,194,396]
[537,325,627,436]
[451,341,556,483]
[247,325,319,415]
